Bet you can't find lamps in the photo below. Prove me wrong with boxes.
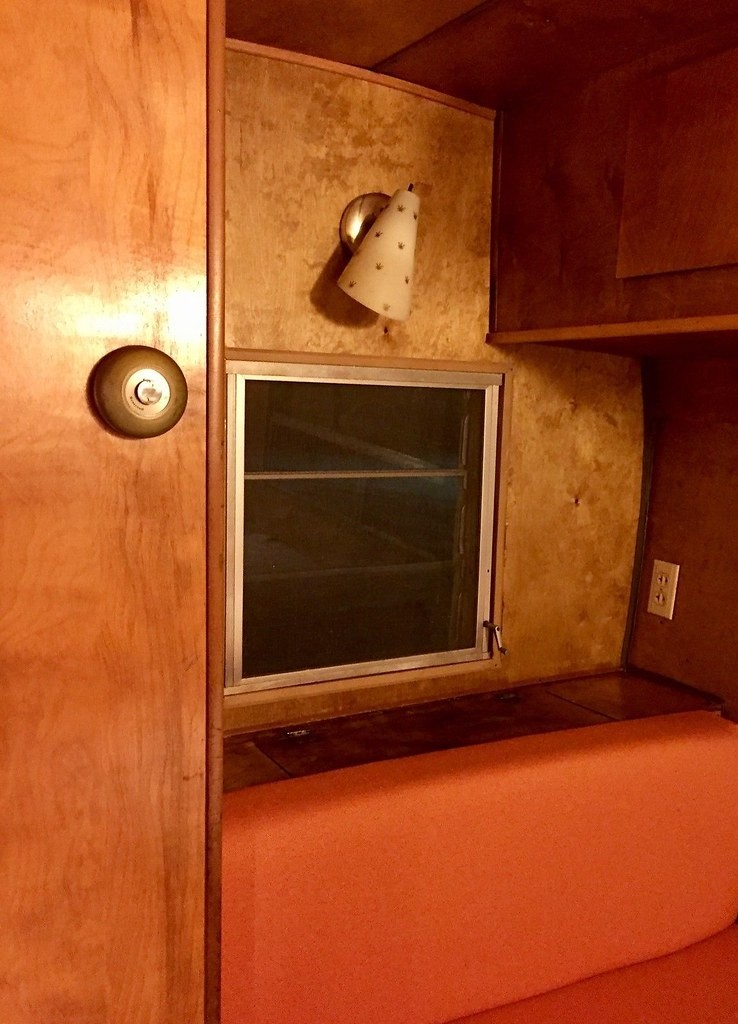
[334,183,421,323]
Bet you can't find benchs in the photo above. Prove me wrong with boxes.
[221,710,738,1024]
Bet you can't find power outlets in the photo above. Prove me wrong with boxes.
[644,559,681,622]
